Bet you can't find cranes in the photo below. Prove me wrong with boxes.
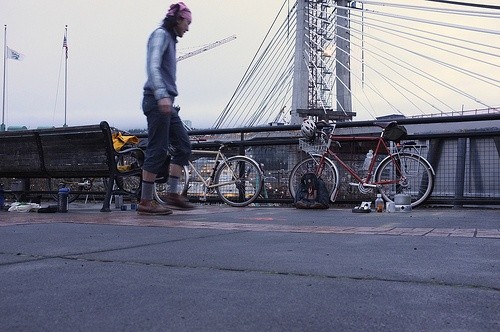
[176,33,237,63]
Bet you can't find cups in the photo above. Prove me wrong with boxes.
[121,205,127,211]
[385,201,395,213]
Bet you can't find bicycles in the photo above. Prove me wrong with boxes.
[153,138,264,207]
[288,120,437,209]
[47,146,143,204]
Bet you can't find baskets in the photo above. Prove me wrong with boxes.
[298,136,327,154]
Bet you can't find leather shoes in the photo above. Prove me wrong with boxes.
[138,201,172,215]
[166,194,195,210]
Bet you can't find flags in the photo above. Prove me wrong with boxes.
[7,48,24,61]
[63,36,68,58]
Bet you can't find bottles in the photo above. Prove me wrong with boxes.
[375,194,384,212]
[57,186,69,213]
[362,149,374,171]
[131,188,137,211]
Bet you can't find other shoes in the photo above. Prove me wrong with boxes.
[296,201,308,208]
[314,202,327,210]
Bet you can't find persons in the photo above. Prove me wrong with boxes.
[136,2,196,215]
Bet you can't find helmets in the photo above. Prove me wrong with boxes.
[301,119,316,138]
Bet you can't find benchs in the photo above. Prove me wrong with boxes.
[0,121,146,213]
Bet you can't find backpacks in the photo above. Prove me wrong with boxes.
[294,173,329,207]
